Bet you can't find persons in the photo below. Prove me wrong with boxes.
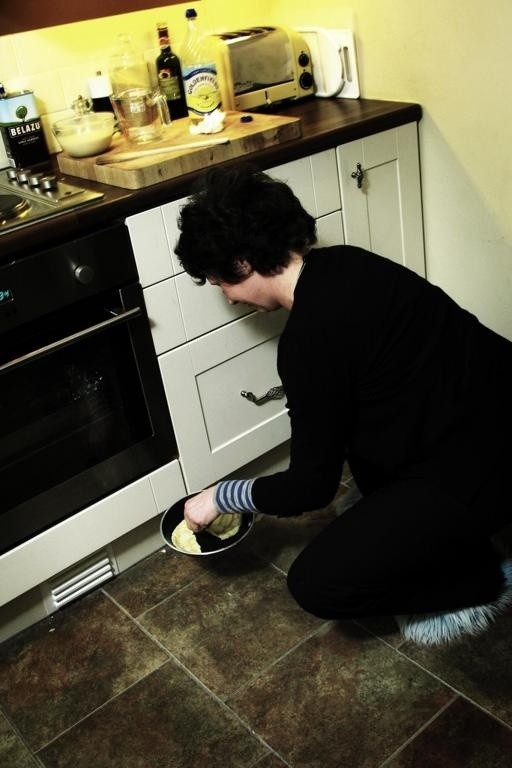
[175,164,512,646]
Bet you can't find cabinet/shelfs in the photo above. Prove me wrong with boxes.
[124,122,426,496]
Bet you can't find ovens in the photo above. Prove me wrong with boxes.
[1,216,182,554]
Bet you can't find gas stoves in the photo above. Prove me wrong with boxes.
[0,167,106,238]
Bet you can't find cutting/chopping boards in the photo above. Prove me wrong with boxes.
[56,107,305,191]
[292,27,362,100]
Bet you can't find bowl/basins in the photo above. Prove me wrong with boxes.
[50,111,115,158]
[160,490,257,558]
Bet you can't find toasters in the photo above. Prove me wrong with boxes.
[206,23,317,112]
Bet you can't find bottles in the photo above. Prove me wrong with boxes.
[156,24,188,119]
[178,9,225,128]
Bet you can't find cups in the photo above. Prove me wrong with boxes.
[110,86,171,147]
